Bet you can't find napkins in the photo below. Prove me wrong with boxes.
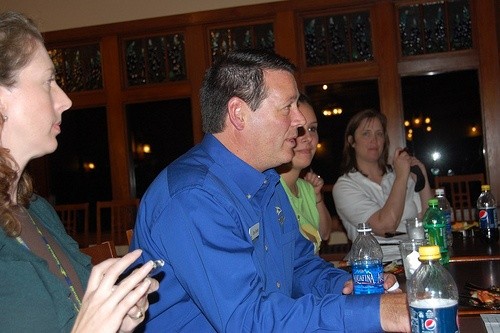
[380,245,402,262]
[373,234,407,244]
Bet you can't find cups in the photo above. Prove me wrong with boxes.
[404,217,425,240]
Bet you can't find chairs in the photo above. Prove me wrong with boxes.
[97,198,138,246]
[56,203,88,247]
[435,174,485,221]
[79,242,117,265]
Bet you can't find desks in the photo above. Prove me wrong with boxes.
[338,229,500,333]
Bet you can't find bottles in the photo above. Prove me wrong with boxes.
[475,184,499,242]
[405,245,461,333]
[422,199,450,270]
[435,188,454,247]
[351,222,385,295]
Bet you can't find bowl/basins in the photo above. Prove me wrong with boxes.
[455,226,476,240]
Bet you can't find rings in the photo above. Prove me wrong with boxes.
[318,175,321,179]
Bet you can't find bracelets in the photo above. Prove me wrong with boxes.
[316,198,322,204]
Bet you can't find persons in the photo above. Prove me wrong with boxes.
[121,47,431,333]
[275,92,332,257]
[0,9,160,333]
[332,110,431,251]
